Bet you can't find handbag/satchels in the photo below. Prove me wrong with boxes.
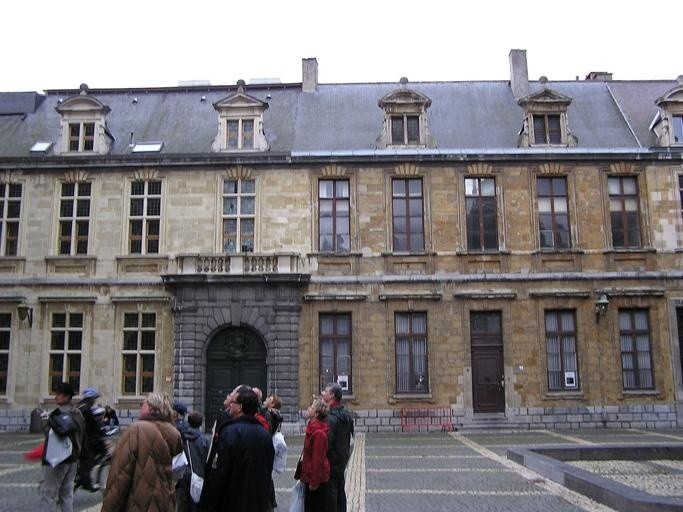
[187,470,204,506]
[44,428,74,469]
[271,430,288,474]
[48,412,79,440]
[292,460,304,481]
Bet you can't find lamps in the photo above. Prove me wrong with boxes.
[594,295,609,323]
[18,306,33,328]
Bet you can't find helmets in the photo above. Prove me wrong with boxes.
[80,387,99,399]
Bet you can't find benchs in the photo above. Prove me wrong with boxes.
[399,405,453,432]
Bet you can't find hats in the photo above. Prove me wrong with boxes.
[51,382,74,396]
[173,400,189,418]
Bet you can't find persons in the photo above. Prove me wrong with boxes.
[76,381,356,512]
[38,382,85,512]
